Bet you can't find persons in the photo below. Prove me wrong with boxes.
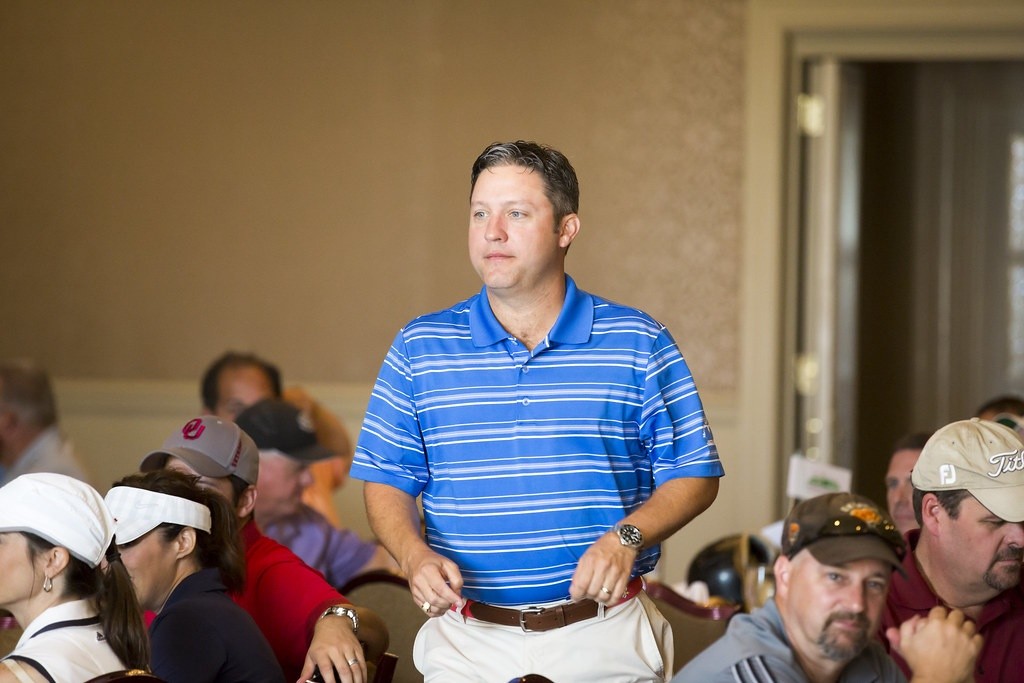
[235,400,405,590]
[0,473,148,683]
[198,348,352,529]
[872,395,1024,683]
[353,141,725,683]
[0,357,102,498]
[669,492,983,683]
[102,469,281,683]
[141,416,368,683]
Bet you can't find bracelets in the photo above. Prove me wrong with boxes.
[321,606,359,634]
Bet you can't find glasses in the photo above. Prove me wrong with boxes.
[787,515,907,562]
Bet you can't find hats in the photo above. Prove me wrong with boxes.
[234,399,335,462]
[0,472,115,568]
[138,415,258,485]
[911,417,1024,523]
[105,485,212,547]
[781,492,911,581]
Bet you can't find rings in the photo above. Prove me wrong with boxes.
[348,659,357,666]
[421,601,431,612]
[601,587,612,595]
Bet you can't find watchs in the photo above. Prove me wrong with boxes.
[604,523,645,551]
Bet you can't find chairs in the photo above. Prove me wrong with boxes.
[300,570,733,683]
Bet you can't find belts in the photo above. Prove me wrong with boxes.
[451,575,643,632]
